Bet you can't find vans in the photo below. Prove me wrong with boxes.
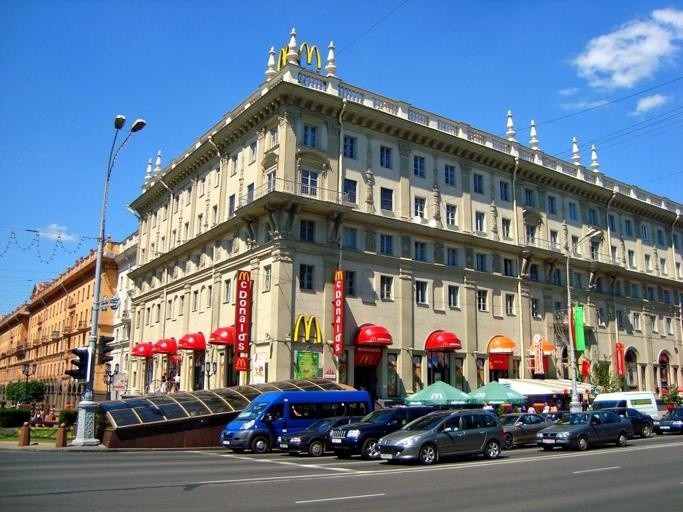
[220,390,373,453]
[592,391,657,418]
[377,409,504,464]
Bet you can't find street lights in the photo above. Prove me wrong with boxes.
[201,362,217,390]
[71,115,146,446]
[21,363,36,403]
[104,363,119,399]
[567,228,601,415]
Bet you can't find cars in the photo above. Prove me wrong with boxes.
[277,416,363,457]
[499,407,653,450]
[654,408,683,435]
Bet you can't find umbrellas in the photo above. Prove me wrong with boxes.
[468,380,528,404]
[404,380,475,406]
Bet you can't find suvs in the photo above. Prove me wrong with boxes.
[329,406,435,460]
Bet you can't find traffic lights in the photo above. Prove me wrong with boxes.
[64,349,88,379]
[97,336,114,364]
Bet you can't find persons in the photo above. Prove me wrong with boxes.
[0,400,74,427]
[159,369,180,393]
[398,414,407,425]
[666,401,680,415]
[483,394,591,416]
[448,420,458,432]
